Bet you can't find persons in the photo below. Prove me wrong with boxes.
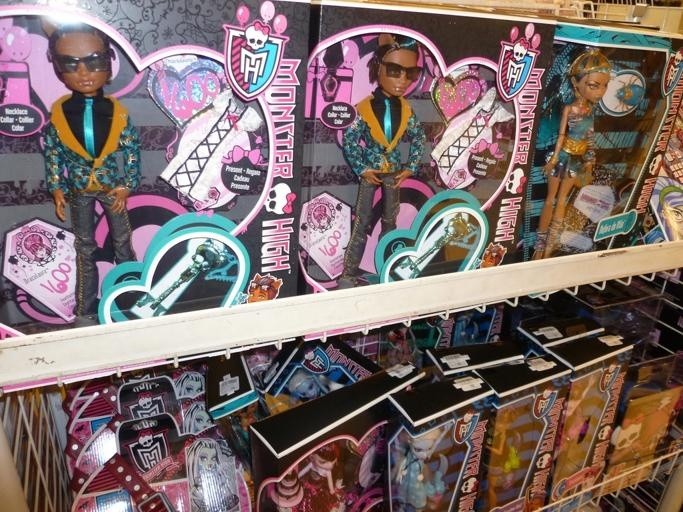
[42,24,139,324]
[532,46,612,259]
[0,286,681,512]
[340,34,425,290]
[553,405,584,488]
[396,428,440,512]
[386,341,411,368]
[289,373,345,405]
[238,416,256,440]
[298,444,345,512]
[487,411,521,510]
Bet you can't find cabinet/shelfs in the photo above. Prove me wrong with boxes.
[0,0,683,512]
